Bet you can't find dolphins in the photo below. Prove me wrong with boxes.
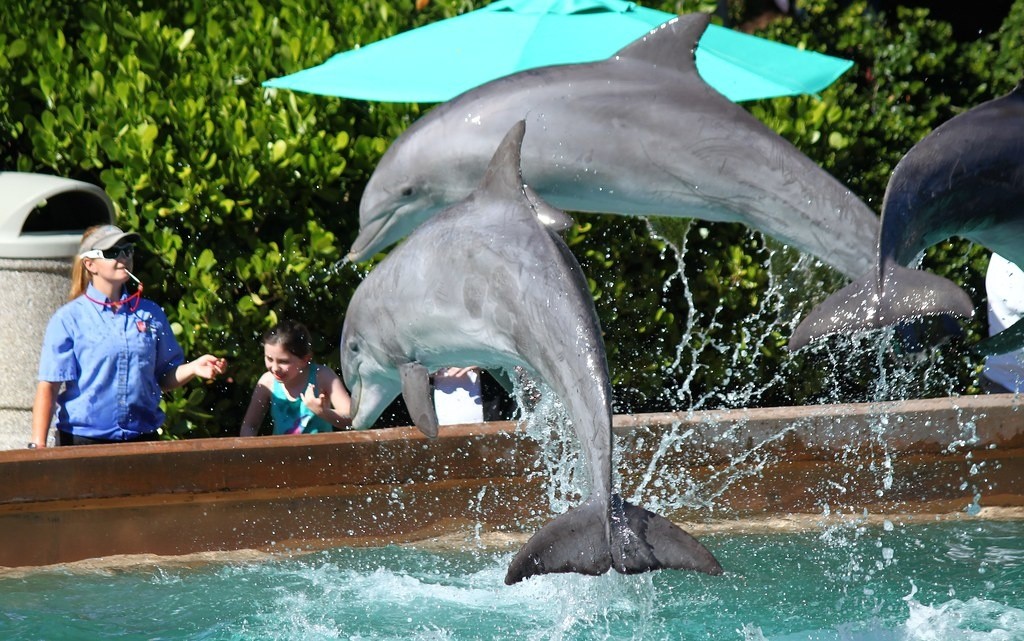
[876,86,1024,304]
[338,120,725,588]
[346,10,978,352]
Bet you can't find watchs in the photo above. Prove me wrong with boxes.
[27,443,46,449]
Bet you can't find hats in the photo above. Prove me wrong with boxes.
[77,225,140,251]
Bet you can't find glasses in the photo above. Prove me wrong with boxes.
[79,243,134,260]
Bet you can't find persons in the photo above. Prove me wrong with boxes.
[428,365,488,426]
[241,319,354,441]
[975,244,1024,394]
[25,224,226,449]
